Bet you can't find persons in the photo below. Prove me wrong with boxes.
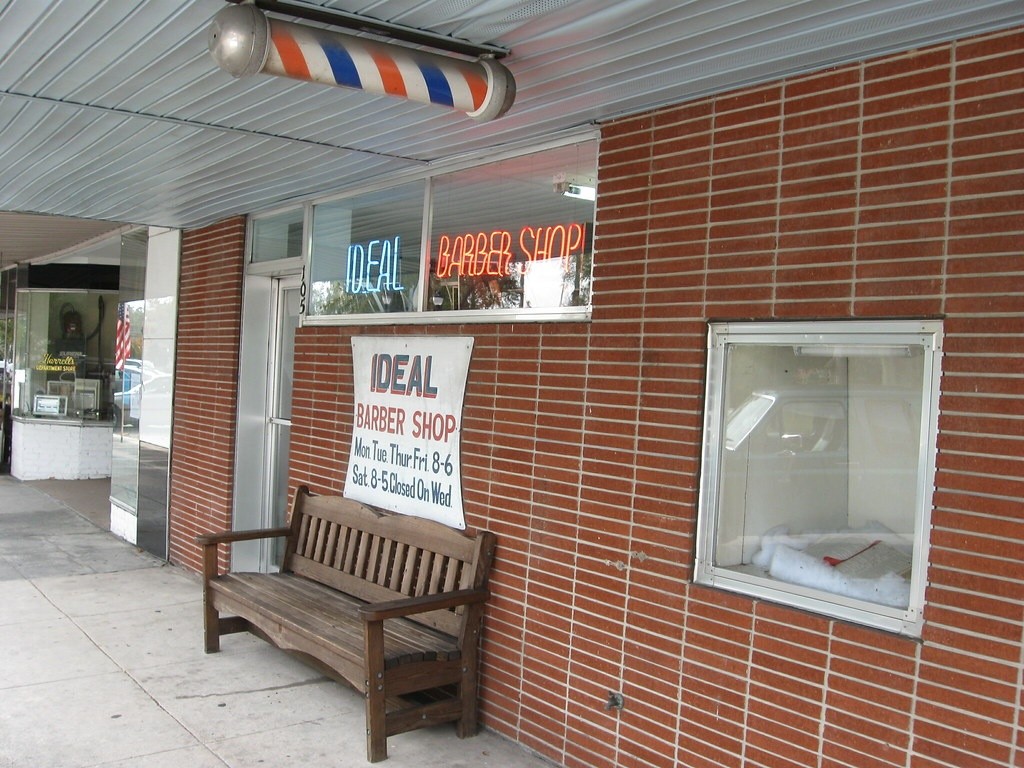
[0,393,13,472]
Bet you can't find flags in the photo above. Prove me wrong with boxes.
[115,303,130,371]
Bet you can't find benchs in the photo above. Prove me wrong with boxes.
[193,484,495,764]
